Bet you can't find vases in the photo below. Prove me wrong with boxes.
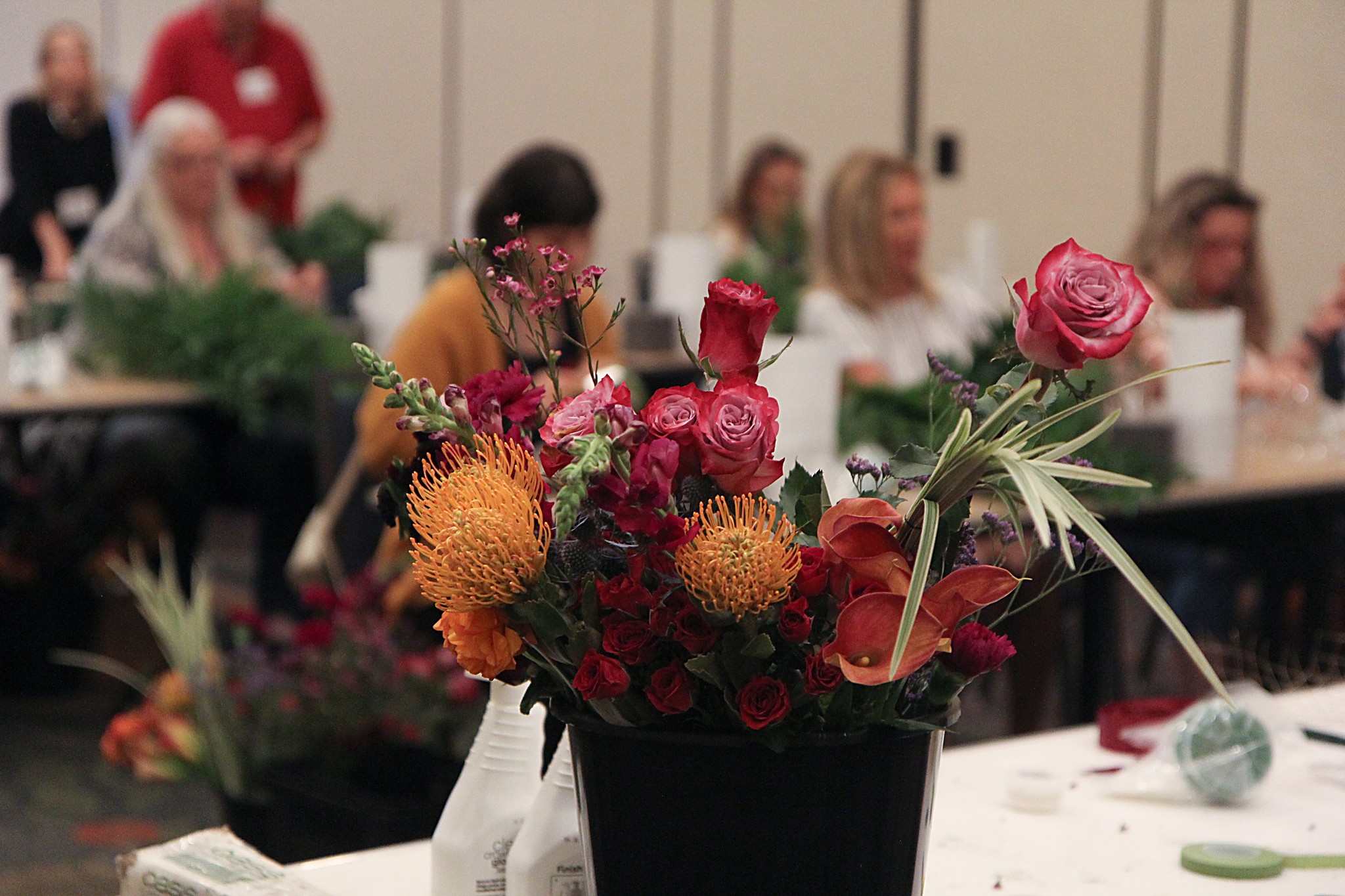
[220,678,490,865]
[566,711,945,896]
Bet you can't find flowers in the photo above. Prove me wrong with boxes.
[68,213,1236,791]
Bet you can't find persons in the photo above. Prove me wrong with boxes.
[62,97,328,367]
[121,1,331,243]
[1105,174,1320,424]
[789,144,993,393]
[1285,266,1345,401]
[697,144,822,338]
[348,147,628,621]
[1,25,117,314]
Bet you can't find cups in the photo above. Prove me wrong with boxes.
[648,234,716,352]
[367,236,424,351]
[1162,306,1244,478]
[760,331,845,479]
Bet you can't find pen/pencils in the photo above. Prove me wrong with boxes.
[1303,726,1345,746]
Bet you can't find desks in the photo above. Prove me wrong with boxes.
[0,372,210,418]
[116,352,1345,896]
